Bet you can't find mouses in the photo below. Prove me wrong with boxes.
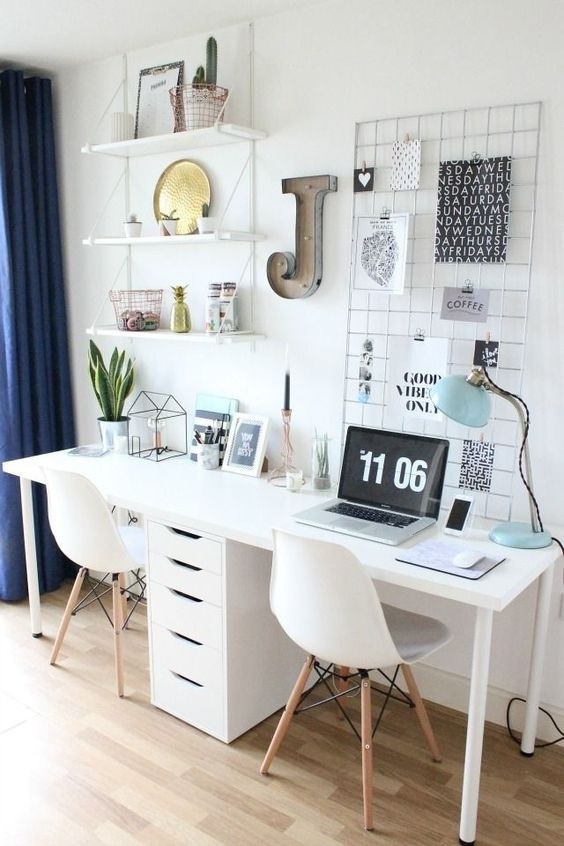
[454,550,486,570]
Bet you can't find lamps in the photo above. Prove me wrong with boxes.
[430,365,552,549]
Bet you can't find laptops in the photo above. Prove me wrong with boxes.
[295,425,451,545]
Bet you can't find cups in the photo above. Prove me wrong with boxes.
[285,468,305,492]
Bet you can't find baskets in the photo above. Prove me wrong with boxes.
[168,84,230,134]
[108,288,164,331]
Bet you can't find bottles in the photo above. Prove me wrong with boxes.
[218,281,239,333]
[207,282,222,334]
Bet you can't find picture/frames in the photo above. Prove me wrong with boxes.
[220,413,269,477]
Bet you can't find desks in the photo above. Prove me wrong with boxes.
[3,444,564,846]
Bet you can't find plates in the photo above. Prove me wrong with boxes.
[152,159,210,235]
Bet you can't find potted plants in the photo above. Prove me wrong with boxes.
[183,35,222,128]
[87,340,134,447]
[310,427,331,488]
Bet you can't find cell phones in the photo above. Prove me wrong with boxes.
[443,495,473,537]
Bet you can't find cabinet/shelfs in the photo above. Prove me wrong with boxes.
[81,22,264,352]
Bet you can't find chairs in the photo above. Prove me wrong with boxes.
[260,529,454,834]
[45,468,146,700]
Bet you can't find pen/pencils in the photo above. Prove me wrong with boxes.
[195,419,223,444]
